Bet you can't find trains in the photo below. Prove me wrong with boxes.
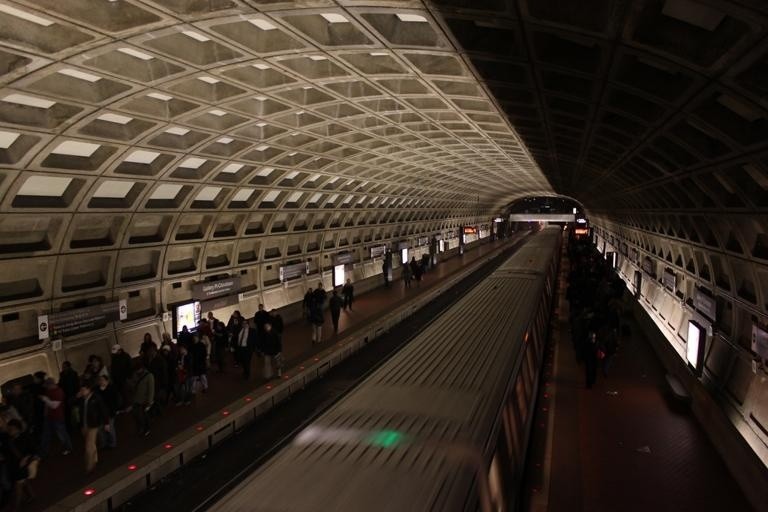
[187,220,563,508]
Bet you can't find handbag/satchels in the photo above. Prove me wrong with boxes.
[26,456,41,480]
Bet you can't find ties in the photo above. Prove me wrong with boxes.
[239,328,246,347]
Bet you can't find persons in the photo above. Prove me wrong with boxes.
[413,263,422,288]
[400,262,412,289]
[301,287,314,315]
[564,230,627,390]
[328,290,345,334]
[306,302,326,345]
[341,277,355,312]
[1,303,285,512]
[419,255,428,275]
[409,256,416,269]
[381,259,386,283]
[312,281,327,315]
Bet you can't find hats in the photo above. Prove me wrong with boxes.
[111,344,121,354]
[162,345,171,352]
